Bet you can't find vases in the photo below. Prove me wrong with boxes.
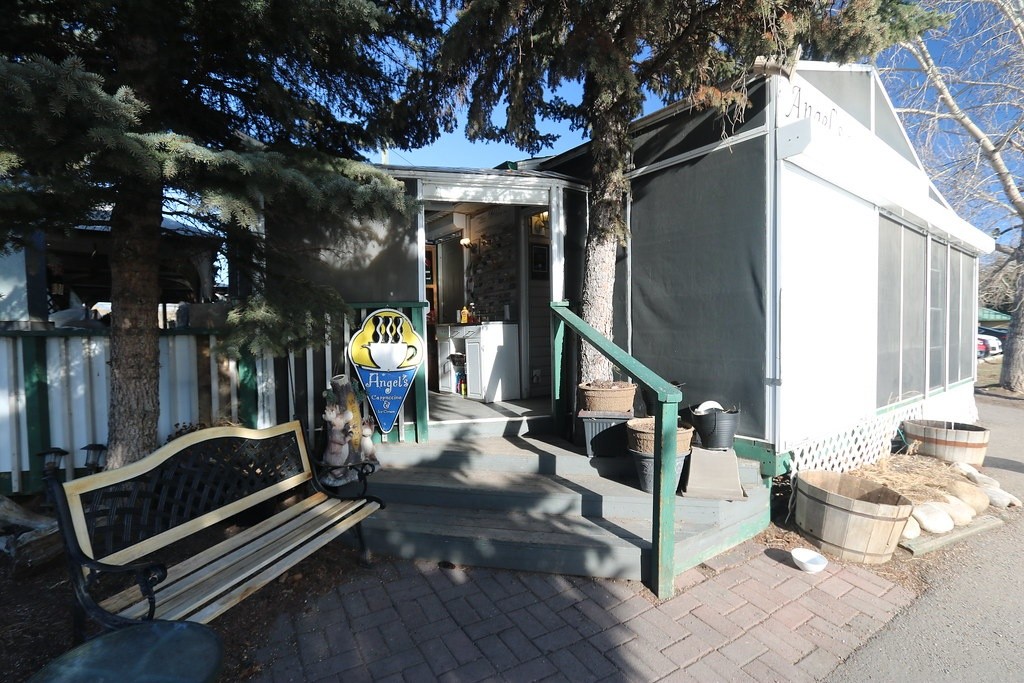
[688,404,740,450]
[578,378,638,412]
[627,417,695,452]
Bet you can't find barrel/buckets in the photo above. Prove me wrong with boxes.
[902,418,990,473]
[796,468,915,564]
[688,400,741,451]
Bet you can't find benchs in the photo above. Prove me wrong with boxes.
[50,415,385,642]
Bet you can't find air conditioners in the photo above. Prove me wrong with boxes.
[426,212,464,240]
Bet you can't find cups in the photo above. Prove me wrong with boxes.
[368,342,417,370]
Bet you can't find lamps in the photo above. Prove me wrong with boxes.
[460,238,478,254]
[36,446,70,472]
[535,211,549,233]
[80,443,107,468]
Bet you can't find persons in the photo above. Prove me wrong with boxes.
[46,273,91,328]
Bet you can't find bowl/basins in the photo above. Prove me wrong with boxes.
[791,548,829,574]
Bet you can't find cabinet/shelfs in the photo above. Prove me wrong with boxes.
[436,324,521,404]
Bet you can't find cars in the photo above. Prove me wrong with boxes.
[976,326,1008,358]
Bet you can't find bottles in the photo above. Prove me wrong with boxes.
[468,302,477,324]
[460,306,469,324]
[504,302,510,321]
